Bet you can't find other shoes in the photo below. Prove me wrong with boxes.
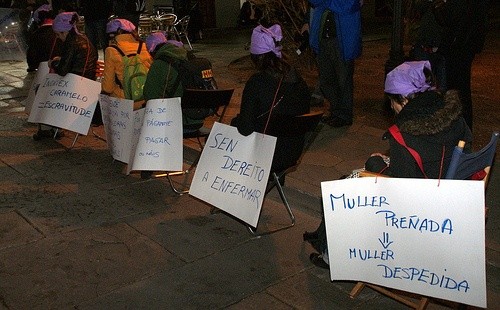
[327,117,353,127]
[322,115,336,123]
[310,253,329,268]
[33,129,56,141]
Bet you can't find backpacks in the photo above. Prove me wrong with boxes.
[160,51,219,119]
[107,39,148,101]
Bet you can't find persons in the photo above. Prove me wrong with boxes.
[231,24,310,171]
[309,0,363,127]
[143,32,204,126]
[429,0,487,130]
[20,0,153,141]
[303,60,473,270]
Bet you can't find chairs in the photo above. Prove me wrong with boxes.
[350,131,500,310]
[53,60,107,149]
[139,10,194,49]
[136,87,236,193]
[207,94,329,225]
[0,22,26,62]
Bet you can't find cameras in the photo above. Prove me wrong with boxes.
[294,32,309,55]
